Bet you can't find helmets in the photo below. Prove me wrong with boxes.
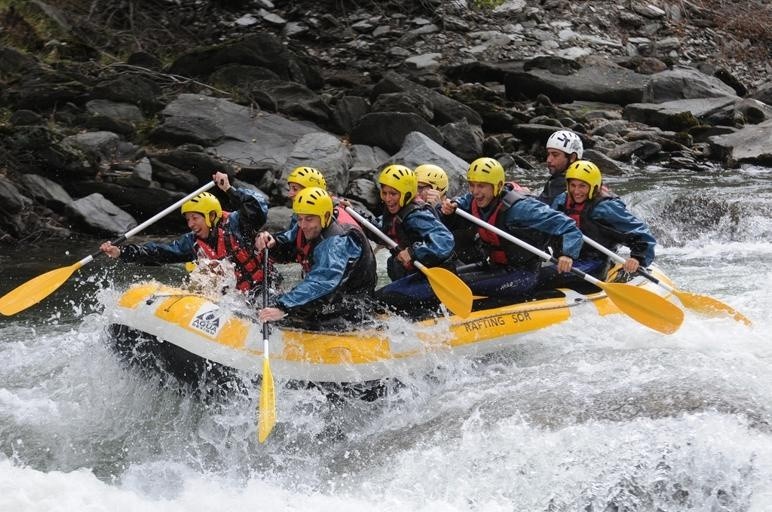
[378,164,449,208]
[287,167,333,230]
[546,130,584,160]
[565,159,602,200]
[467,157,505,198]
[181,190,223,229]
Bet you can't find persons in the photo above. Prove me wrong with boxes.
[99,130,657,331]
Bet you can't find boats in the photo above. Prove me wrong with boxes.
[108,261,684,419]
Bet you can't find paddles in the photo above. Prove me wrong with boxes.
[454,207,753,333]
[258,246,275,442]
[0,177,216,316]
[333,195,473,320]
